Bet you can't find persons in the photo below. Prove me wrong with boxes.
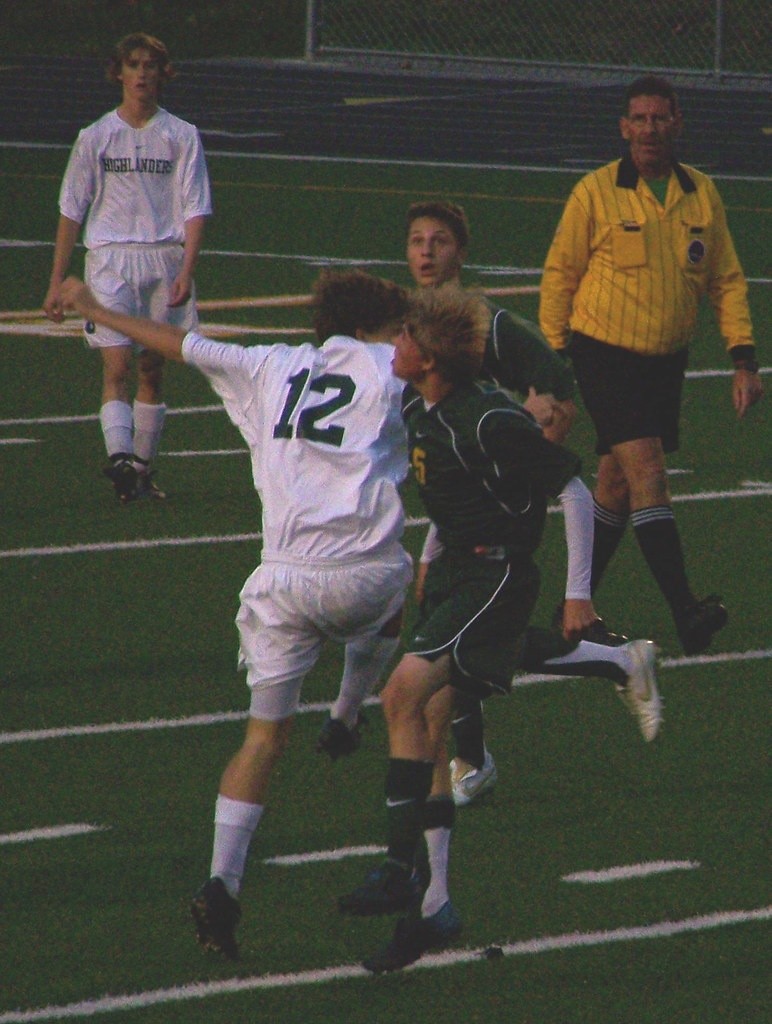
[61,198,663,973]
[42,31,213,502]
[540,75,765,659]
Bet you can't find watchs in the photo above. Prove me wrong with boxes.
[735,358,759,373]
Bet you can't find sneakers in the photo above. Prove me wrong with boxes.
[613,640,662,743]
[448,740,498,807]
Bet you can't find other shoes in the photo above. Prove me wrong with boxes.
[187,878,244,963]
[677,602,727,655]
[108,453,136,498]
[317,718,361,756]
[338,850,421,915]
[551,612,626,646]
[362,900,462,971]
[133,468,165,500]
